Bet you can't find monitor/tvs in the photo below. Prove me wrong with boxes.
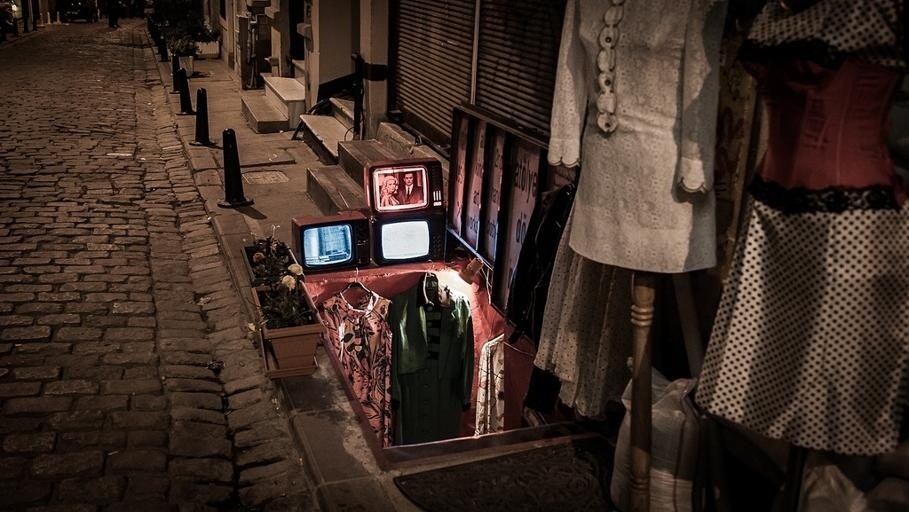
[364,156,445,218]
[292,210,371,273]
[373,216,447,267]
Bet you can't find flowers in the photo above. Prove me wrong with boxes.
[252,224,289,264]
[261,264,317,326]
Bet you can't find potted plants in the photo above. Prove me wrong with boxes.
[165,27,200,77]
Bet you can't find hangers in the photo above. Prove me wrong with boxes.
[340,268,371,295]
[423,259,438,282]
[566,165,575,199]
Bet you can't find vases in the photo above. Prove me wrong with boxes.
[241,242,299,282]
[251,280,323,380]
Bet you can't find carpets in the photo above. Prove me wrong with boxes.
[393,436,622,511]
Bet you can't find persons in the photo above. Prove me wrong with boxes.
[396,172,423,205]
[380,176,400,208]
